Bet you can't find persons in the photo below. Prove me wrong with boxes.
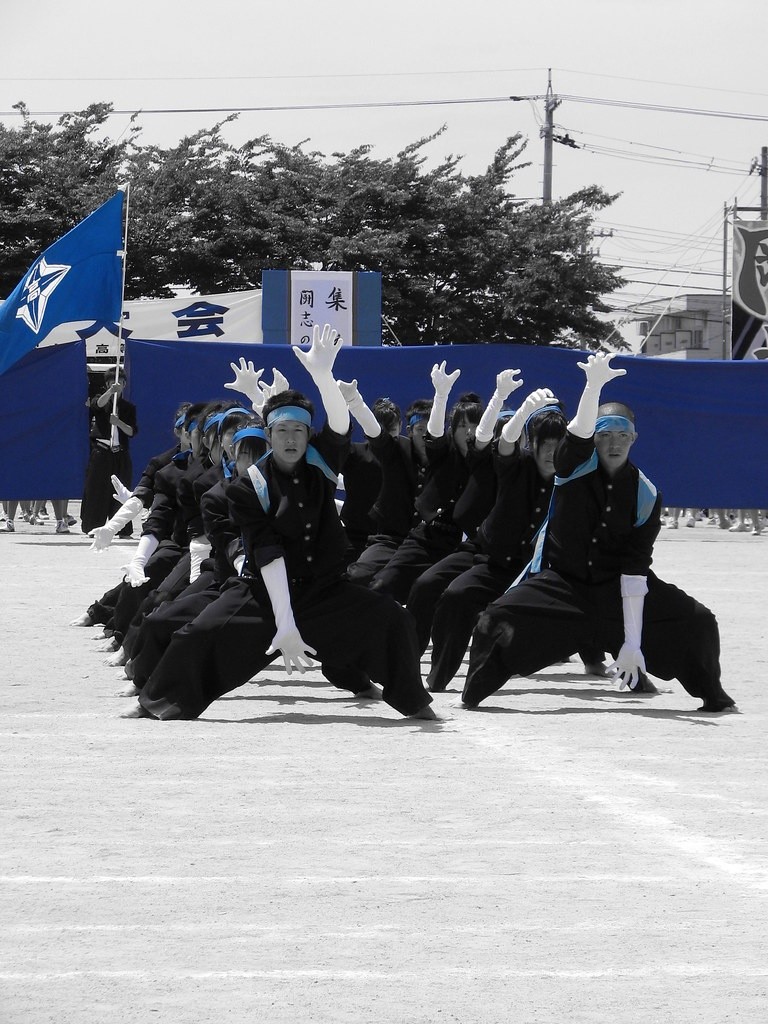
[70,324,739,720]
[0,499,77,533]
[80,364,137,539]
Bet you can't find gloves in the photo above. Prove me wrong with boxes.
[88,497,140,553]
[427,360,461,437]
[224,357,264,405]
[251,367,289,420]
[604,574,647,691]
[335,379,381,439]
[120,535,159,587]
[259,559,317,674]
[566,351,626,439]
[291,324,350,435]
[502,387,559,443]
[189,542,213,583]
[109,474,150,517]
[232,554,246,576]
[475,369,524,442]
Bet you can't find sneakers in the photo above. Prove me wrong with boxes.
[55,520,70,534]
[18,511,44,525]
[37,509,49,520]
[0,518,14,532]
[660,508,768,535]
[0,509,8,521]
[66,515,77,526]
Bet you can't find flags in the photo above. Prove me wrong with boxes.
[0,184,127,374]
